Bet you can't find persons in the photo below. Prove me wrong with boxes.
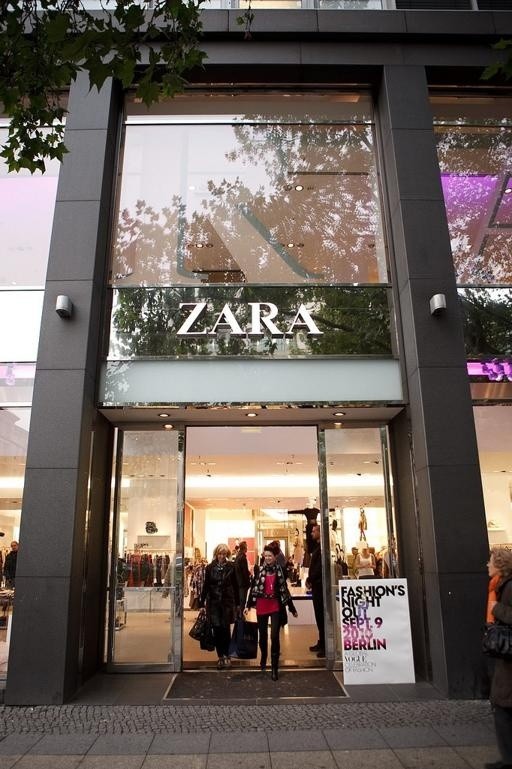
[201,524,377,682]
[483,547,510,767]
[4,542,18,588]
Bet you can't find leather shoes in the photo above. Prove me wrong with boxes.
[217,658,232,670]
[272,666,278,682]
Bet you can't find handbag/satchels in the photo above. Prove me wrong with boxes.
[229,619,259,659]
[482,624,512,659]
[200,625,216,651]
[189,618,209,642]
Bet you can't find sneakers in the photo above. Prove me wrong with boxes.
[310,645,320,651]
[317,650,324,657]
